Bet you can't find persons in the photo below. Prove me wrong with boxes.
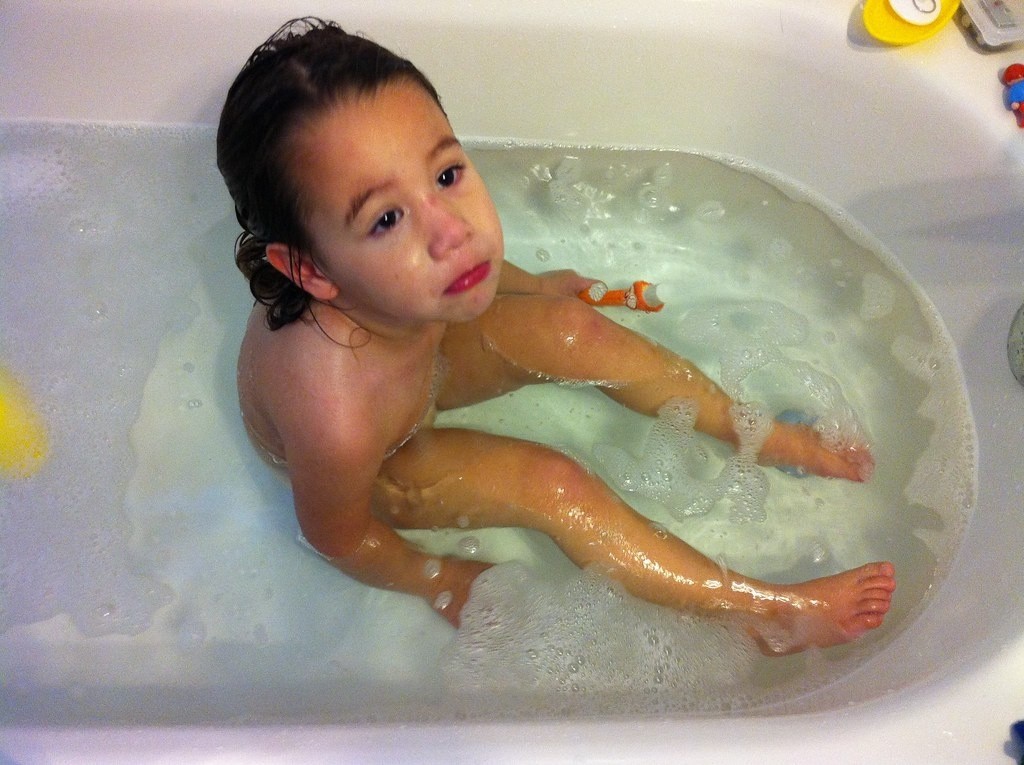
[215,17,894,658]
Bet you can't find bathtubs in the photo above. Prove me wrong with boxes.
[1,2,1024,763]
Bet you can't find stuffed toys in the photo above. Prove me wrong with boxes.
[1003,64,1024,129]
